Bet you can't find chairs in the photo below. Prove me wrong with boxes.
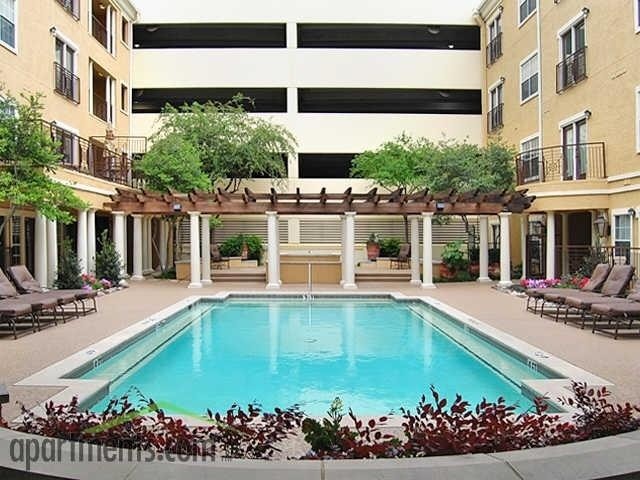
[211,245,230,269]
[525,263,640,340]
[0,264,97,340]
[390,243,410,269]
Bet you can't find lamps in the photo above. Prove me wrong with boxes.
[535,220,546,238]
[595,216,611,238]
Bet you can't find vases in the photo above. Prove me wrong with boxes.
[469,263,500,280]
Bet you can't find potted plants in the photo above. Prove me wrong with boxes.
[219,231,264,257]
[366,232,384,260]
[439,241,463,279]
